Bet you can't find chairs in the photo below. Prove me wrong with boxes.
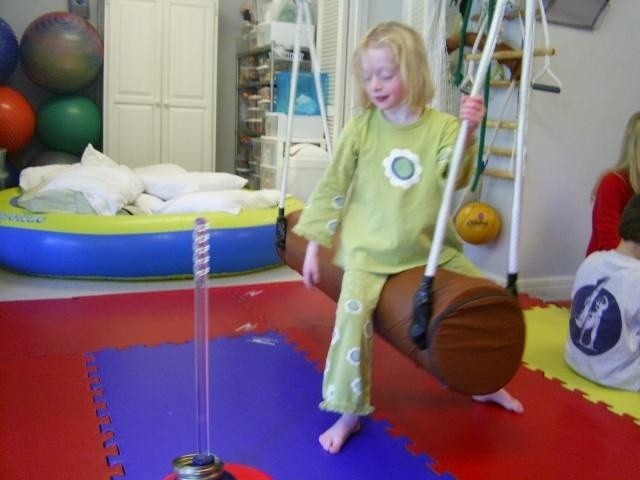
[101,0,221,172]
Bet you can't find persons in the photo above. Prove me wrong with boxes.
[289,19,525,457]
[583,111,639,259]
[562,194,640,394]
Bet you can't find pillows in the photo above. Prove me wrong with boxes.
[15,142,293,217]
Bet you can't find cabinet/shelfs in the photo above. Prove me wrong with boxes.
[234,39,316,190]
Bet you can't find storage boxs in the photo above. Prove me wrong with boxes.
[238,19,315,51]
[257,70,330,190]
